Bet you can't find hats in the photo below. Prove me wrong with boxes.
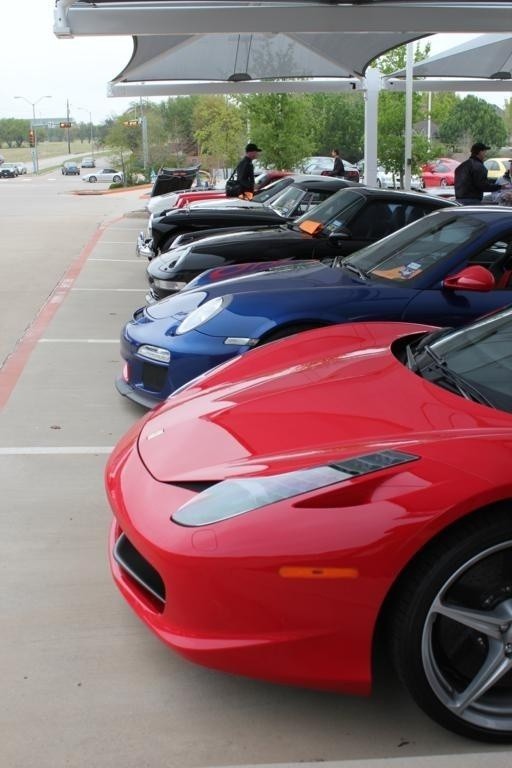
[247,144,261,151]
[472,143,490,151]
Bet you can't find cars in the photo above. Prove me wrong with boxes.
[292,157,360,182]
[0,156,5,165]
[81,157,95,168]
[484,158,512,178]
[61,162,80,176]
[81,167,123,183]
[419,158,461,187]
[0,162,19,178]
[100,305,512,744]
[14,162,28,176]
[358,159,416,187]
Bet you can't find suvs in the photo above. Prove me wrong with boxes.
[113,205,512,410]
[134,174,367,262]
[143,186,460,310]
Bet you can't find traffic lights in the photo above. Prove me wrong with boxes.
[60,122,71,128]
[28,131,35,148]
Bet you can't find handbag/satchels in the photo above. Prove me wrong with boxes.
[226,180,242,197]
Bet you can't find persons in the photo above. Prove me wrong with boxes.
[492,159,512,206]
[236,144,262,198]
[328,148,344,180]
[454,143,512,206]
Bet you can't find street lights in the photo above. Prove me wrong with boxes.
[12,94,53,175]
[79,107,96,162]
[123,118,149,168]
